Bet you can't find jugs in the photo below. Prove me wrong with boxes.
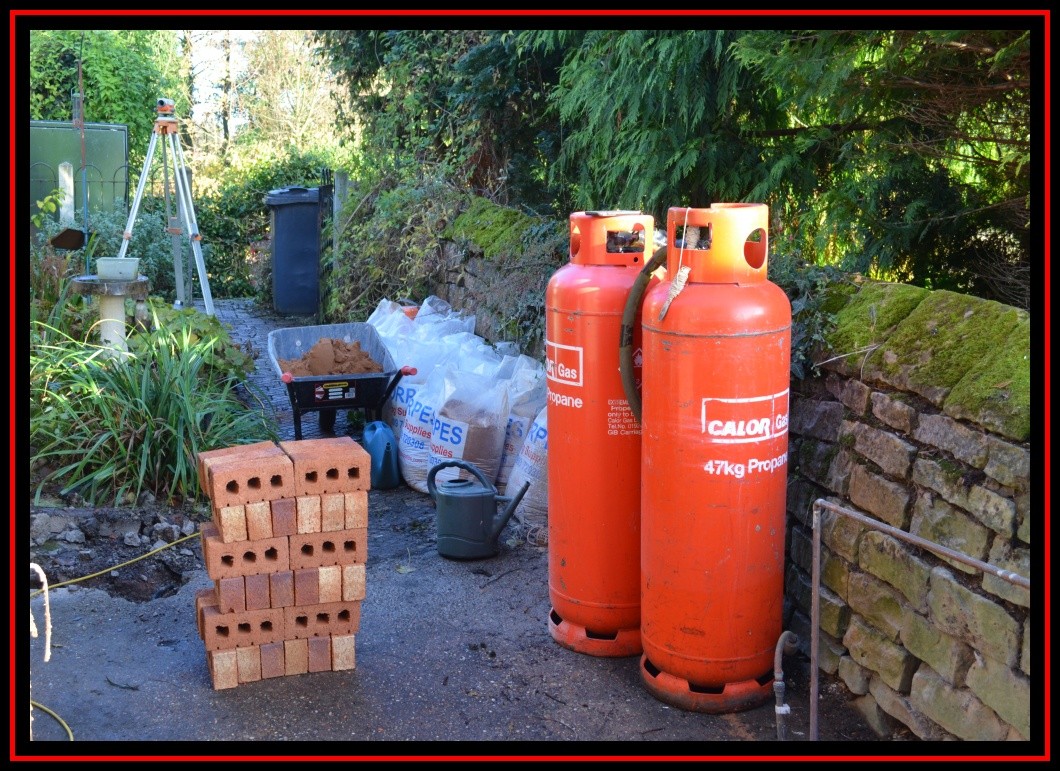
[427,459,531,559]
[362,420,399,488]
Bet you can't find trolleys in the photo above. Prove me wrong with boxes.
[267,321,418,440]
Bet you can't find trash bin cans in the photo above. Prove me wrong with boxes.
[261,187,320,315]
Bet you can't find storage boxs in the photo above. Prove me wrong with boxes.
[95,257,141,281]
